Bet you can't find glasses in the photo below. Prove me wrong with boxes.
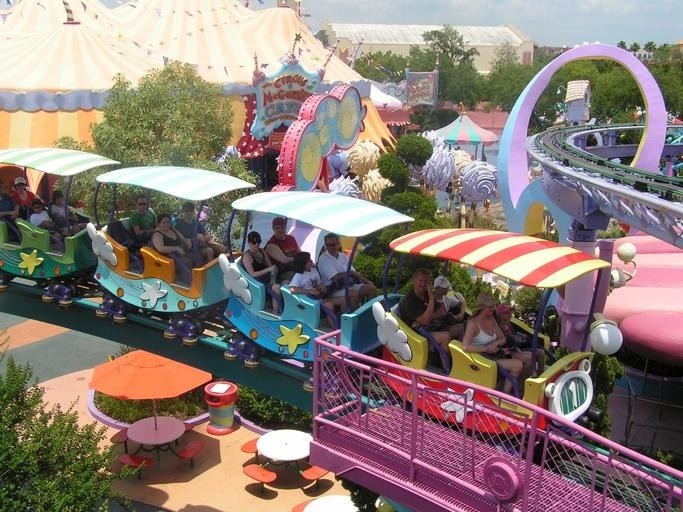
[16,183,24,186]
[138,203,146,205]
[251,241,262,244]
[35,207,41,209]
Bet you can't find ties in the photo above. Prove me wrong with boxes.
[497,304,515,315]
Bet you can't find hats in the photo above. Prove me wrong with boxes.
[477,295,496,307]
[273,217,286,225]
[14,177,26,185]
[434,276,451,289]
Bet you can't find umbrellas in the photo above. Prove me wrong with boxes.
[90,348,213,433]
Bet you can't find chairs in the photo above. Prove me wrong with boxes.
[107,220,143,274]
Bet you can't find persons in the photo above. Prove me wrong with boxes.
[130,195,226,285]
[397,270,545,395]
[0,178,87,250]
[241,218,379,331]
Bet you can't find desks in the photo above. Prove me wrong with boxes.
[126,416,185,461]
[255,429,313,476]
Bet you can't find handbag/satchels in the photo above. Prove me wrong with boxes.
[334,275,361,288]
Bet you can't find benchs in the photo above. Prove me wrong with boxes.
[240,437,329,495]
[109,423,202,494]
